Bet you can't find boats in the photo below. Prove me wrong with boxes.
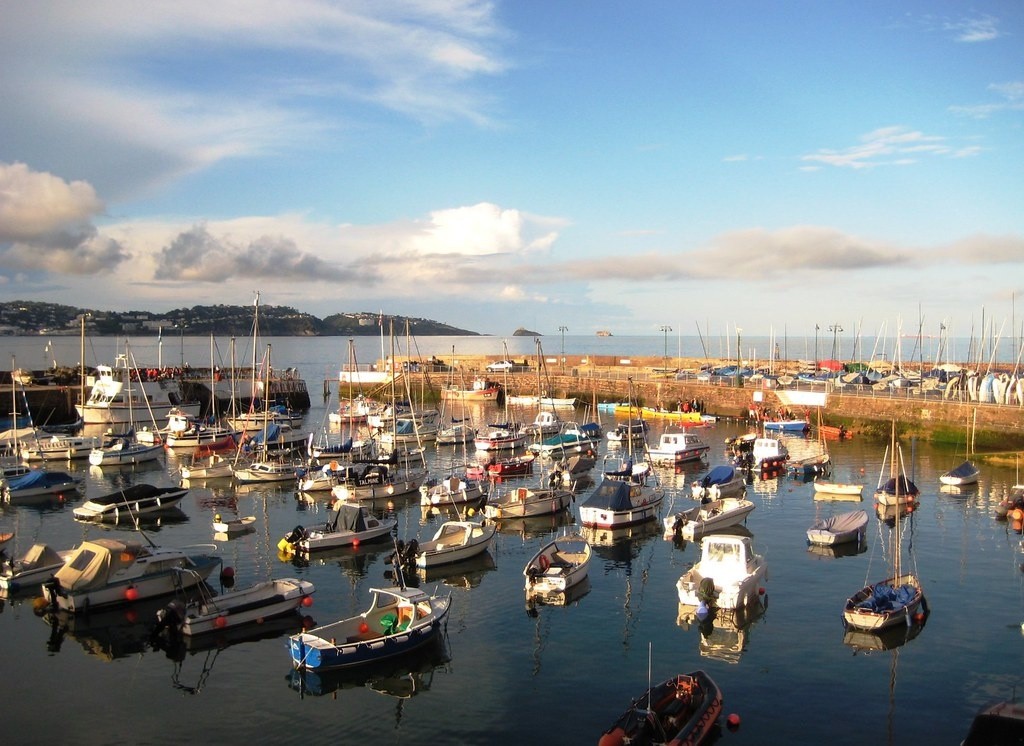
[1,290,649,500]
[765,419,807,432]
[748,438,789,471]
[213,516,257,533]
[0,549,80,591]
[874,473,917,507]
[418,475,484,508]
[938,461,980,486]
[73,482,187,527]
[523,531,591,591]
[484,488,573,519]
[155,577,316,636]
[578,478,667,530]
[286,500,397,555]
[789,454,829,475]
[411,521,499,568]
[645,432,709,465]
[814,479,863,495]
[661,498,755,540]
[2,469,85,502]
[289,585,454,673]
[840,573,922,631]
[806,509,869,546]
[598,669,722,746]
[675,532,766,609]
[42,538,223,613]
[599,403,717,424]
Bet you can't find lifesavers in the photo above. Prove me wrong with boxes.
[330,460,337,471]
[539,554,551,570]
